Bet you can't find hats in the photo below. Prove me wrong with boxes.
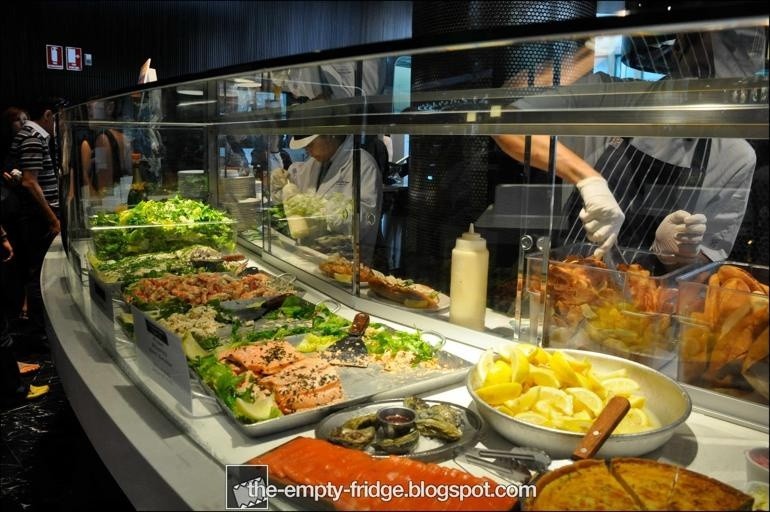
[289,99,333,150]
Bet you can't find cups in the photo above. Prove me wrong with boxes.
[746,447,769,493]
[376,403,417,438]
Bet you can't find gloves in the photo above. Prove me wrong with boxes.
[579,176,626,260]
[287,192,320,217]
[649,209,707,266]
[585,9,631,55]
[270,167,290,190]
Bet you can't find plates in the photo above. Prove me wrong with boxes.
[218,175,256,202]
[178,170,209,199]
[366,288,451,313]
[316,398,484,460]
[223,199,260,231]
[317,267,384,287]
[218,166,248,176]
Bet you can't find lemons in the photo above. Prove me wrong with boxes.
[473,341,651,434]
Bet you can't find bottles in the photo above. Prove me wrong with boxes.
[127,153,148,209]
[281,177,309,240]
[447,221,491,328]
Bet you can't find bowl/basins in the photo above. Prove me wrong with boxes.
[466,348,693,461]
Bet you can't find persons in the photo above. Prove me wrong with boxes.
[289,135,384,267]
[2,107,30,135]
[99,163,101,164]
[487,136,758,299]
[251,135,292,175]
[0,225,14,262]
[80,91,136,201]
[4,96,61,353]
[501,28,770,89]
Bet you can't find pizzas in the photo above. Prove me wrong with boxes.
[521,457,757,512]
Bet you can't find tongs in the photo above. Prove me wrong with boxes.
[599,238,636,304]
[452,446,552,486]
